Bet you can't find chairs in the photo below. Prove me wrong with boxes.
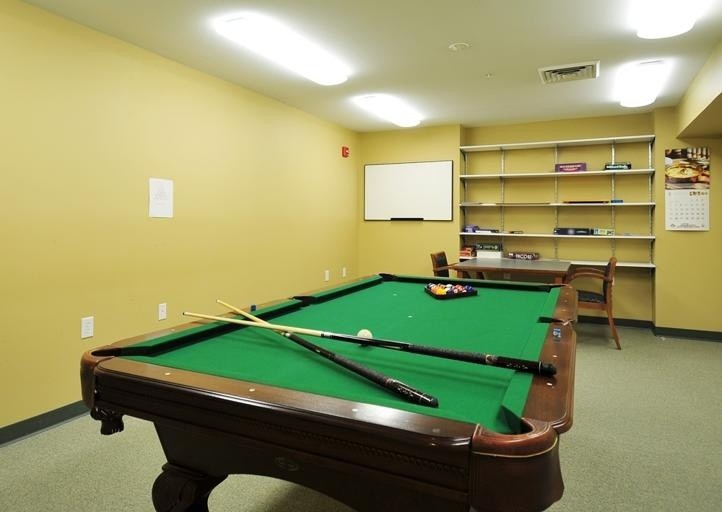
[430,252,457,277]
[563,257,622,350]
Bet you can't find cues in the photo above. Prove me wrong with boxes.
[216,300,439,409]
[182,312,556,376]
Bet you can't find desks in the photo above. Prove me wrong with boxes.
[80,273,578,512]
[452,257,571,284]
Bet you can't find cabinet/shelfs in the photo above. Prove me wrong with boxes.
[458,135,656,269]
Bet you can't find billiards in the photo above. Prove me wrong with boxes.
[427,283,473,295]
[357,329,372,339]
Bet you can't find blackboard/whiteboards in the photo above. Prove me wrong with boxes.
[364,160,452,221]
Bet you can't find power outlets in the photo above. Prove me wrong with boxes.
[159,303,166,321]
[81,317,94,339]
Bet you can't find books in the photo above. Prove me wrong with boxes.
[460,243,499,257]
[475,229,499,233]
[501,230,523,234]
